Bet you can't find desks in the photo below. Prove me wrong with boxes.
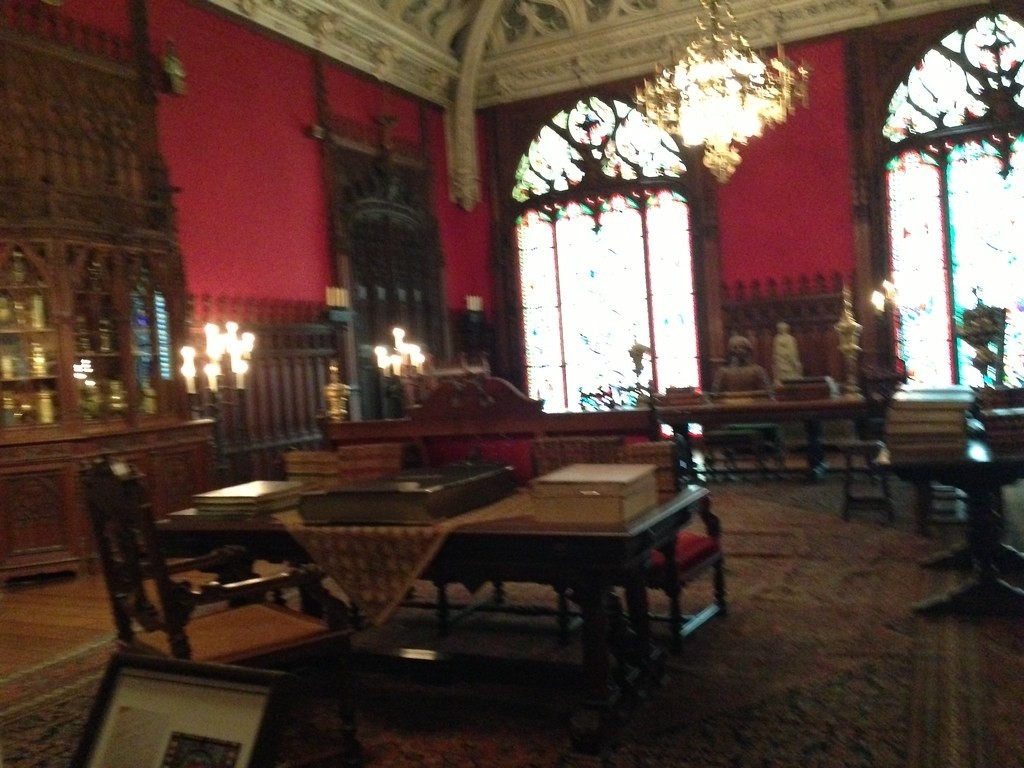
[655,395,873,483]
[875,438,1023,618]
[154,480,711,708]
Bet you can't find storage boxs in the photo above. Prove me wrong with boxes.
[534,464,660,530]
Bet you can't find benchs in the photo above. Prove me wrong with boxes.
[326,375,682,638]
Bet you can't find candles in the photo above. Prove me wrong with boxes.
[374,328,425,376]
[870,268,930,316]
[180,319,254,392]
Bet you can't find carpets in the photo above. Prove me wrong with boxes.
[3,485,1024,768]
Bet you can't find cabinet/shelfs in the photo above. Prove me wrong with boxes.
[0,223,220,583]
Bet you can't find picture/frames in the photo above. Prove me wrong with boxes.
[71,651,285,768]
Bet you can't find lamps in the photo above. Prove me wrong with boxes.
[634,1,811,183]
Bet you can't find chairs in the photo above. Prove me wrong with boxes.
[705,354,783,482]
[77,458,360,768]
[313,419,502,634]
[533,435,730,651]
